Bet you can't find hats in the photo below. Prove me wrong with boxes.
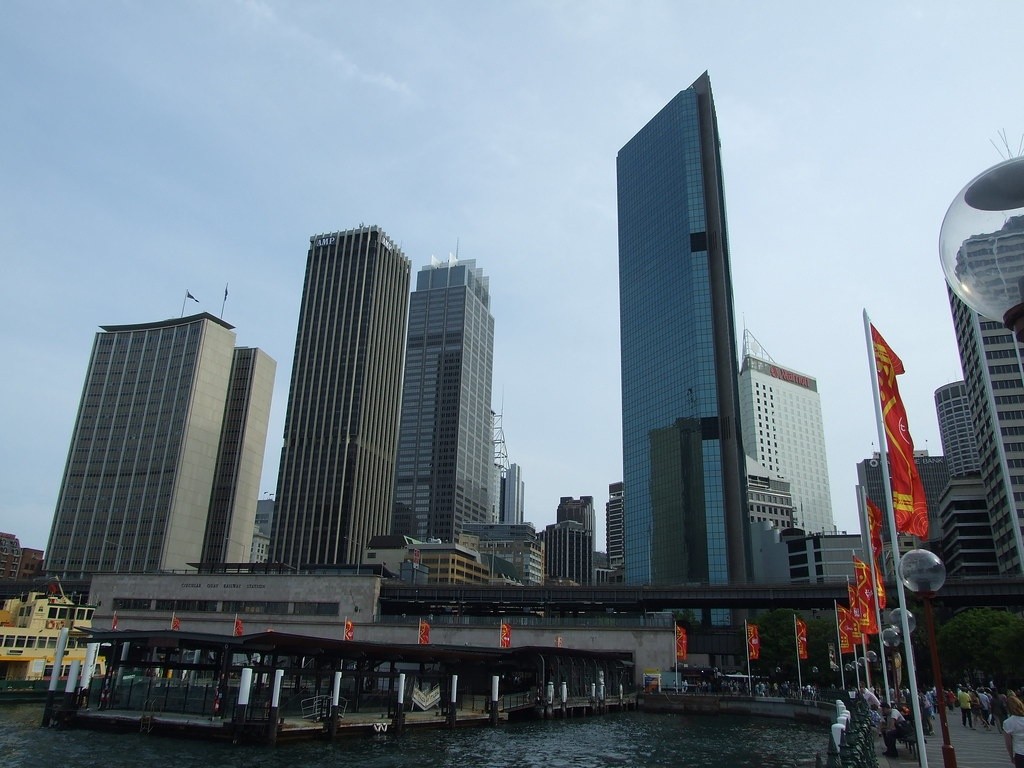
[959,684,962,687]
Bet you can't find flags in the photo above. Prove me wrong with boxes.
[172,614,180,631]
[836,493,886,653]
[112,611,119,631]
[746,624,759,660]
[234,618,244,636]
[501,623,511,648]
[797,619,807,659]
[345,618,354,640]
[419,621,430,644]
[869,323,929,542]
[675,626,687,661]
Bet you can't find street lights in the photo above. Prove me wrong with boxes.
[343,537,362,576]
[104,539,123,574]
[225,537,246,561]
[898,548,957,768]
[844,607,925,767]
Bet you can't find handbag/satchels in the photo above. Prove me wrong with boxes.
[976,701,985,711]
[954,698,960,707]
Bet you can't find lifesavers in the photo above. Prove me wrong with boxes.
[45,620,55,630]
[55,621,65,630]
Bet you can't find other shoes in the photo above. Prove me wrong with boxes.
[986,728,991,731]
[970,728,976,730]
[882,751,897,756]
[929,731,935,736]
[962,726,967,728]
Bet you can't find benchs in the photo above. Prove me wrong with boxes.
[901,736,927,760]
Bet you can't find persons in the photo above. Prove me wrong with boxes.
[1003,695,1024,768]
[672,677,855,701]
[880,702,907,758]
[855,679,1024,735]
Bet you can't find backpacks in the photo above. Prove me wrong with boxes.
[894,719,914,739]
